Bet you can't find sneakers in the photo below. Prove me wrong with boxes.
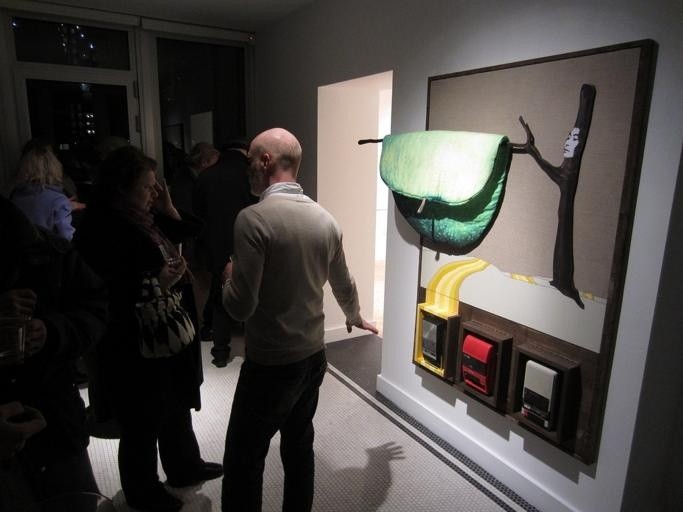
[167,461,222,487]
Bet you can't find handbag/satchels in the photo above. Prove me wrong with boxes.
[134,277,199,360]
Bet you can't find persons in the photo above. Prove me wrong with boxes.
[187,139,259,369]
[218,127,379,512]
[175,142,219,188]
[69,144,223,512]
[0,194,99,512]
[8,137,86,242]
[0,395,50,464]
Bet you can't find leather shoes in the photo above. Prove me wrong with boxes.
[211,360,227,367]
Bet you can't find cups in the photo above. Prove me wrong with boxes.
[4,297,34,360]
[0,324,27,364]
[158,242,182,267]
